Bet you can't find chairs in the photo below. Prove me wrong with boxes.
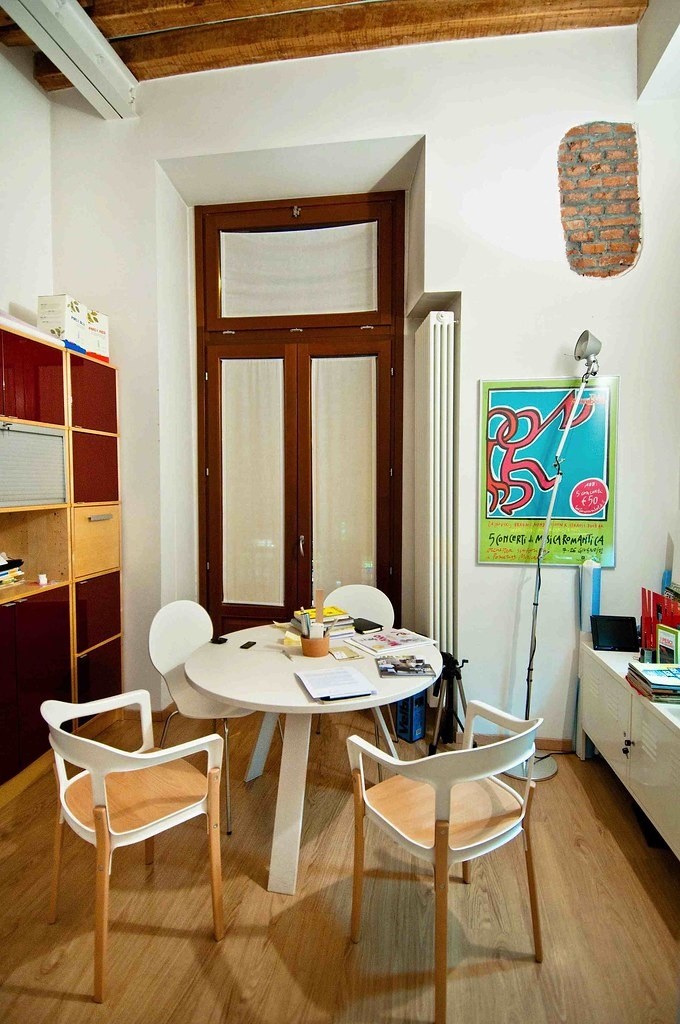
[346,699,543,1024]
[39,689,227,1004]
[314,584,399,784]
[148,598,283,836]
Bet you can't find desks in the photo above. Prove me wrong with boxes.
[183,624,444,895]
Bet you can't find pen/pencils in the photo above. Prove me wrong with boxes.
[324,619,338,636]
[282,649,294,662]
[301,607,311,638]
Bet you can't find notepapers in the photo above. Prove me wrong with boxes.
[284,631,301,645]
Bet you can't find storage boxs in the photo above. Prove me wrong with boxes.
[395,688,428,744]
[37,293,110,358]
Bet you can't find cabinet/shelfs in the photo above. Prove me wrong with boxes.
[0,322,126,810]
[578,640,680,862]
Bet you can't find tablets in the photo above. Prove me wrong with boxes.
[592,614,639,652]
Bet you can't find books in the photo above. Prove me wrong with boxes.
[290,606,355,639]
[0,559,25,590]
[319,693,371,704]
[352,618,383,634]
[658,582,680,620]
[626,662,680,703]
[343,628,437,656]
[656,624,680,664]
[375,655,436,678]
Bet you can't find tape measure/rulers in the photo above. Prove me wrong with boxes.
[316,589,323,622]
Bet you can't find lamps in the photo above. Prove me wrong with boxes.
[507,330,602,783]
